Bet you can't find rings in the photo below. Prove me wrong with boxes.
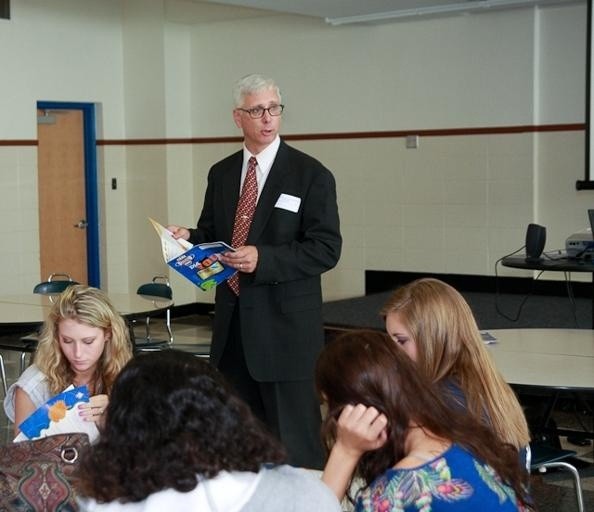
[100,408,104,415]
[239,262,242,269]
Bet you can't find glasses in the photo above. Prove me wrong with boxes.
[234,105,284,119]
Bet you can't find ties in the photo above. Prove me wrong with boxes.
[227,157,258,297]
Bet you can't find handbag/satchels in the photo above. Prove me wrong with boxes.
[0,433,89,512]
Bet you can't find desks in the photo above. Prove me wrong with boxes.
[1,322,44,398]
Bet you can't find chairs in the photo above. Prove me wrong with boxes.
[30,272,81,364]
[137,275,173,353]
[526,438,586,512]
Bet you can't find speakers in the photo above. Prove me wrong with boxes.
[524,224,546,263]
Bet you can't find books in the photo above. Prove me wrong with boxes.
[149,217,241,292]
[18,383,102,447]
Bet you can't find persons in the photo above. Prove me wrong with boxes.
[3,285,135,443]
[165,73,342,471]
[314,329,532,512]
[76,349,345,512]
[379,276,530,487]
